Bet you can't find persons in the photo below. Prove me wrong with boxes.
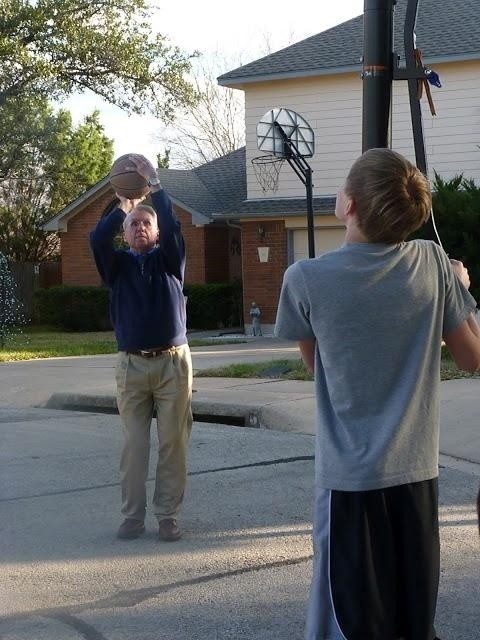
[89,154,193,541]
[250,302,263,336]
[275,148,480,640]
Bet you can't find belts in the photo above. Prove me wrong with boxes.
[130,346,178,358]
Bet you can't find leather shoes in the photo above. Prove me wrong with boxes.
[118,519,145,539]
[159,519,181,541]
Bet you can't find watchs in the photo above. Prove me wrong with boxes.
[148,177,160,187]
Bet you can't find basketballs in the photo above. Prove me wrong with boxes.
[110,154,152,199]
